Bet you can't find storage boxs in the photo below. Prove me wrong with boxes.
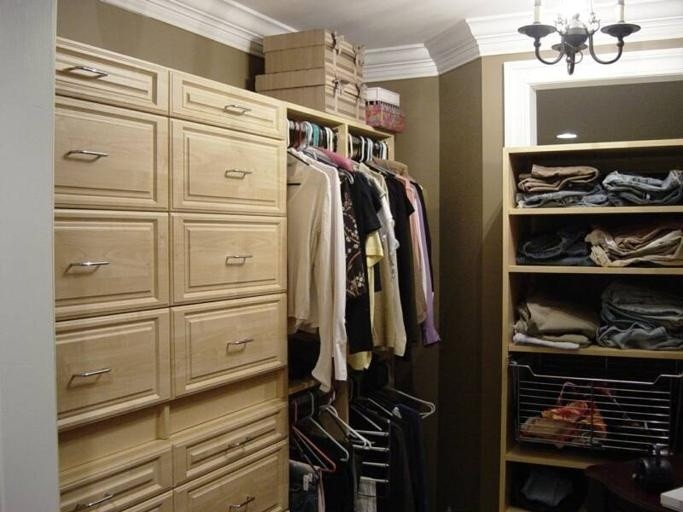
[366,104,406,132]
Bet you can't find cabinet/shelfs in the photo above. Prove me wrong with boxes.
[499,139,682,512]
[54,35,290,511]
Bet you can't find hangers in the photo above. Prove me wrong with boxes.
[288,119,423,187]
[287,364,437,484]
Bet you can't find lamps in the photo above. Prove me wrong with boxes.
[518,1,642,74]
